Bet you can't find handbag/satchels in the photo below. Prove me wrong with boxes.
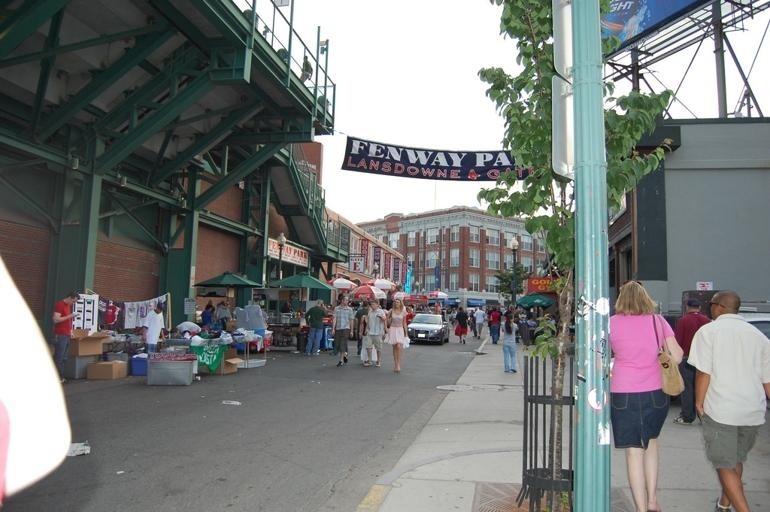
[657,350,688,397]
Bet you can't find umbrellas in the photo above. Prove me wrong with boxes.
[194,271,265,358]
[268,270,450,319]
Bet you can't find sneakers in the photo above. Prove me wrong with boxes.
[375,361,382,368]
[458,334,481,345]
[672,416,693,426]
[363,361,373,367]
[503,368,518,373]
[343,356,348,364]
[336,361,344,368]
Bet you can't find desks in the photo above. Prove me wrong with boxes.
[247,339,268,368]
[167,338,247,369]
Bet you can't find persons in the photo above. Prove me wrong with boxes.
[51,291,81,385]
[202,298,232,339]
[675,297,712,425]
[687,292,770,512]
[168,321,202,340]
[607,280,685,512]
[141,301,164,357]
[279,291,559,374]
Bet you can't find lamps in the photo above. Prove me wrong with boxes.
[70,155,80,170]
[119,175,129,188]
[179,197,188,209]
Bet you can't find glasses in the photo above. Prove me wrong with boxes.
[709,300,727,310]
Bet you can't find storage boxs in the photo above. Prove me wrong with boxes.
[84,361,118,379]
[130,352,148,378]
[64,327,110,358]
[146,357,194,386]
[107,351,130,375]
[113,360,129,378]
[64,351,98,380]
[224,348,244,375]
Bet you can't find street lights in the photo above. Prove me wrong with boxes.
[371,262,381,280]
[510,235,519,306]
[414,280,421,296]
[276,232,286,280]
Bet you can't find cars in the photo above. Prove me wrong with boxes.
[739,311,770,339]
[408,314,450,345]
